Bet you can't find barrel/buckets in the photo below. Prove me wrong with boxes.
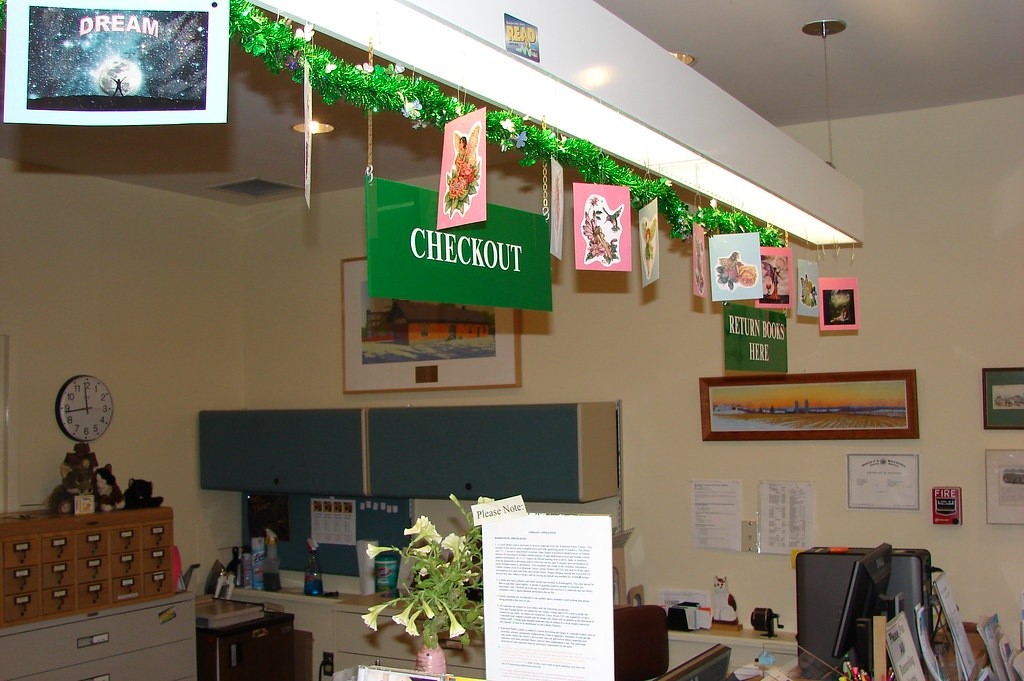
[375,558,398,592]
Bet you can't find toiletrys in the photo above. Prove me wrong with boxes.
[251,537,267,590]
[240,545,252,588]
[226,546,241,588]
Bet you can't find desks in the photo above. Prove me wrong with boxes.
[194,611,294,681]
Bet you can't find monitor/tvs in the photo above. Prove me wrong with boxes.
[652,643,732,681]
[831,542,892,674]
[4,1,229,128]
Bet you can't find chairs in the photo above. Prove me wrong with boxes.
[611,605,670,681]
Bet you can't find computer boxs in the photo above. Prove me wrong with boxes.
[796,546,934,670]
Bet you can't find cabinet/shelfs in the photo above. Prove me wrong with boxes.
[1,592,198,681]
[0,504,174,628]
[334,594,484,678]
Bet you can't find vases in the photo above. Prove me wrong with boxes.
[417,629,447,676]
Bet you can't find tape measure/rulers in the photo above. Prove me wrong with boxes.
[872,616,888,681]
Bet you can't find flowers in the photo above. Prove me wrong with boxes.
[361,493,497,640]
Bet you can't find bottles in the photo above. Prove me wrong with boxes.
[304,546,324,595]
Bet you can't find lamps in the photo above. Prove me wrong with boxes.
[243,0,866,253]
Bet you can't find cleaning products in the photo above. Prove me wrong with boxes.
[304,537,324,596]
[262,528,282,592]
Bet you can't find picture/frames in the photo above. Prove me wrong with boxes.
[339,255,521,395]
[698,370,920,442]
[981,366,1024,430]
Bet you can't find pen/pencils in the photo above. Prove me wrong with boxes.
[838,653,895,681]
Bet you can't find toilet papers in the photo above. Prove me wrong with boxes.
[356,539,379,597]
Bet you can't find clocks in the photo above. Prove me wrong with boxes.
[54,375,116,443]
